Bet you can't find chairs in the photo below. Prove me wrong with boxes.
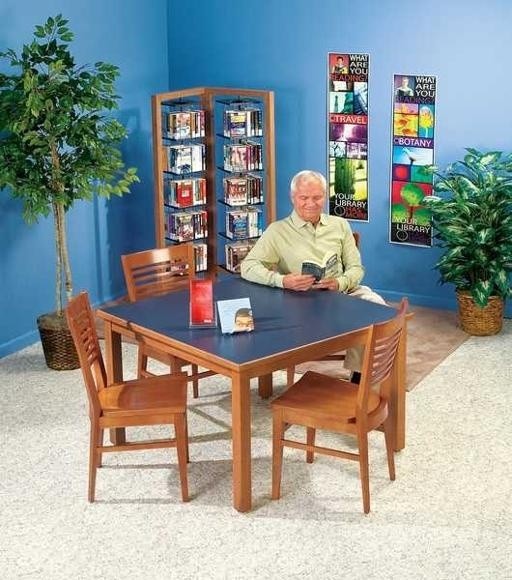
[66,290,190,503]
[122,245,208,399]
[279,232,363,390]
[270,297,410,515]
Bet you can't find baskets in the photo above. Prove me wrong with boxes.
[454,286,504,336]
[35,312,82,371]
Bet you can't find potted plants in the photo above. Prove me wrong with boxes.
[418,147,512,337]
[0,14,140,371]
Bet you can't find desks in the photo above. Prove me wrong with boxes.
[98,272,415,512]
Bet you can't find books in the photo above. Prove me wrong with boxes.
[189,278,215,325]
[166,142,207,175]
[223,106,264,138]
[222,173,264,207]
[225,206,265,241]
[300,247,340,281]
[165,207,209,243]
[165,177,207,208]
[167,108,207,140]
[224,240,256,274]
[216,296,255,334]
[223,139,263,173]
[169,241,208,276]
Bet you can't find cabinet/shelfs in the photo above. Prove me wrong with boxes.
[151,86,276,283]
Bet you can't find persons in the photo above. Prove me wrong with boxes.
[395,77,414,96]
[239,169,391,385]
[331,56,348,74]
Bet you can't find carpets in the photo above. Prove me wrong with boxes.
[272,301,472,393]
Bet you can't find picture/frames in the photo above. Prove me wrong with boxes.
[325,52,373,224]
[388,72,437,247]
